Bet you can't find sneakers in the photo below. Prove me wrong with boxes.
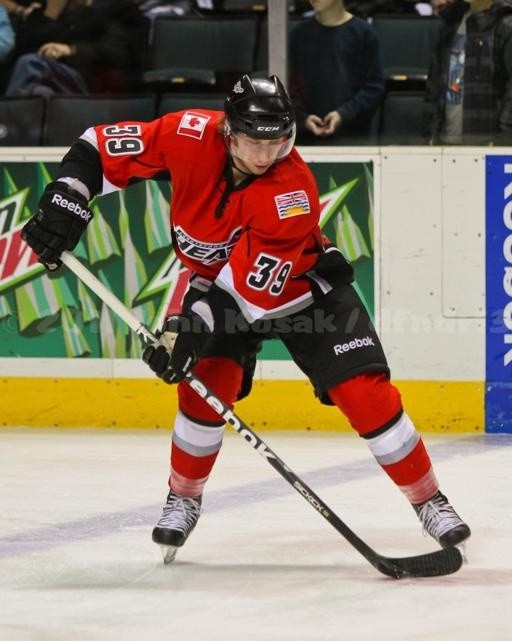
[152,488,204,547]
[409,490,472,551]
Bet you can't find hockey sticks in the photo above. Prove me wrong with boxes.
[57,251,463,581]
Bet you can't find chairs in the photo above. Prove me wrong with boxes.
[0,0,511,148]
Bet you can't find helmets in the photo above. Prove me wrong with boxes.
[221,72,299,142]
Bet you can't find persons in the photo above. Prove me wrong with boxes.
[420,1,511,146]
[1,0,70,57]
[5,1,135,99]
[286,1,381,146]
[17,70,471,549]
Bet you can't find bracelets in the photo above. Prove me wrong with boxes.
[18,6,26,18]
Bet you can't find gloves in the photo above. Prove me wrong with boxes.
[18,180,94,270]
[140,308,212,385]
